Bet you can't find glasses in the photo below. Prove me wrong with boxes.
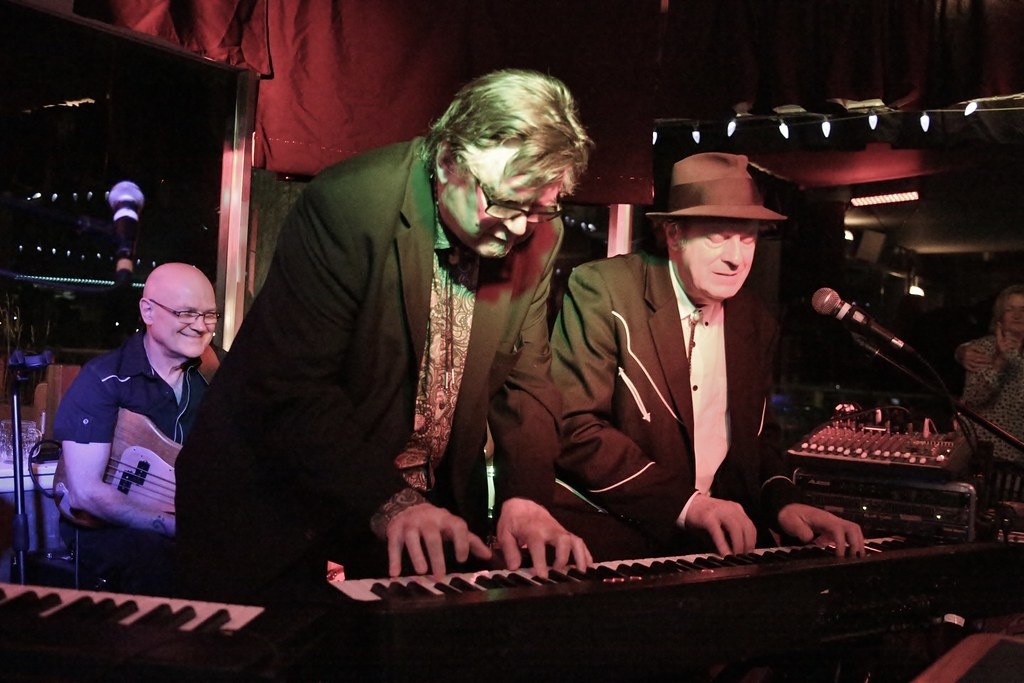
[149,298,219,324]
[474,178,564,224]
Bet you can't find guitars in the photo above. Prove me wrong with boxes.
[52,404,185,529]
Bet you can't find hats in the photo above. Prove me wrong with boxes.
[646,151,787,221]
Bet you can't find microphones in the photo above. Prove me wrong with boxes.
[109,181,144,284]
[812,288,916,355]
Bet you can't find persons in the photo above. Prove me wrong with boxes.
[53,263,228,597]
[173,71,592,607]
[954,284,1024,498]
[550,153,863,558]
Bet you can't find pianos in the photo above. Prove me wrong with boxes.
[318,530,1024,683]
[0,581,318,683]
